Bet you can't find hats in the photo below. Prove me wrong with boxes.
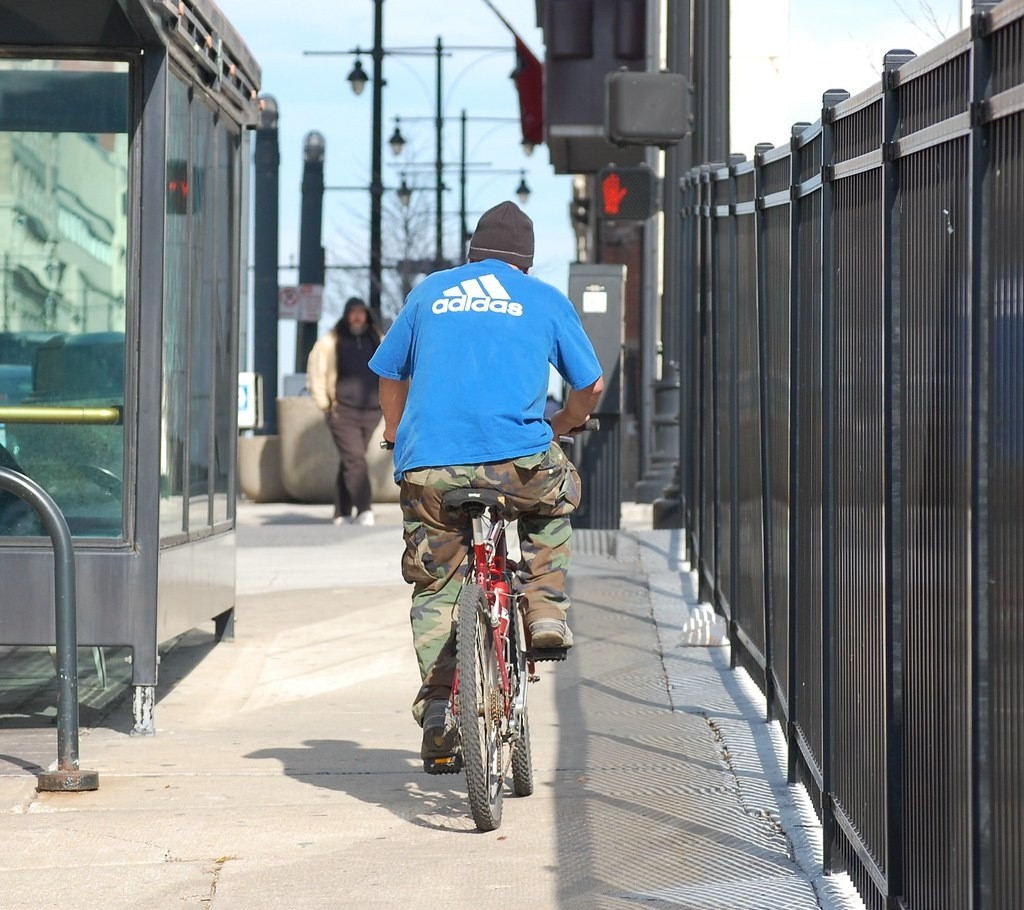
[467,202,534,268]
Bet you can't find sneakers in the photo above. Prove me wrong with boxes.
[420,699,460,760]
[530,619,573,649]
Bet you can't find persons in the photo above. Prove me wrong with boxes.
[368,200,607,767]
[307,297,384,526]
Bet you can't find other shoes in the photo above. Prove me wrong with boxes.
[333,510,374,527]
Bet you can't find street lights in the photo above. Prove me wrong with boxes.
[299,0,529,331]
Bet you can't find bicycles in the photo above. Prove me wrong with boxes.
[370,415,601,833]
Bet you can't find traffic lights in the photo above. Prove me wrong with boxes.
[591,168,657,217]
